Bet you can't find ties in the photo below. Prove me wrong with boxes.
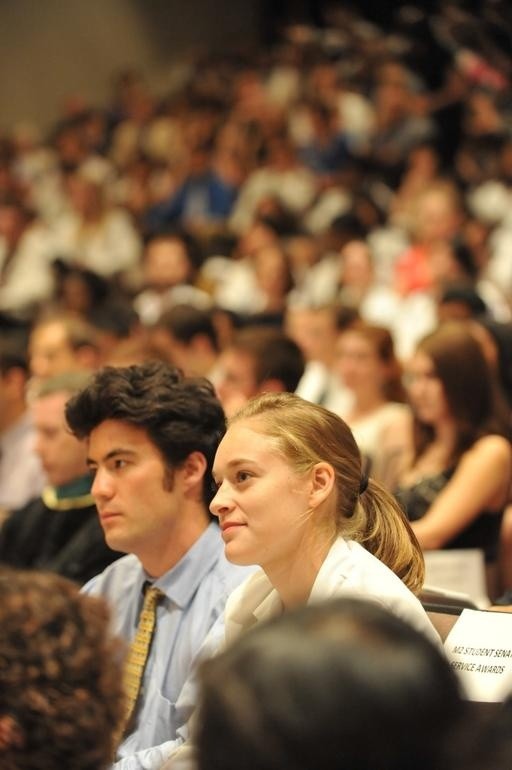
[109,583,167,763]
